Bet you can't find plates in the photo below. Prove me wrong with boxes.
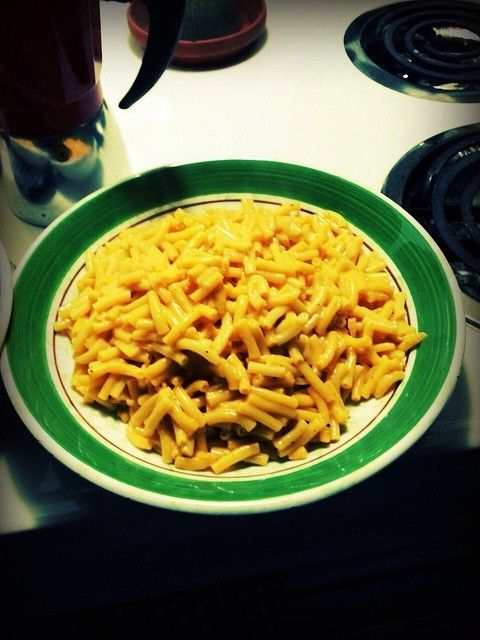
[1,159,466,516]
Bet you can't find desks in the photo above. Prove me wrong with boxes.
[0,1,479,532]
